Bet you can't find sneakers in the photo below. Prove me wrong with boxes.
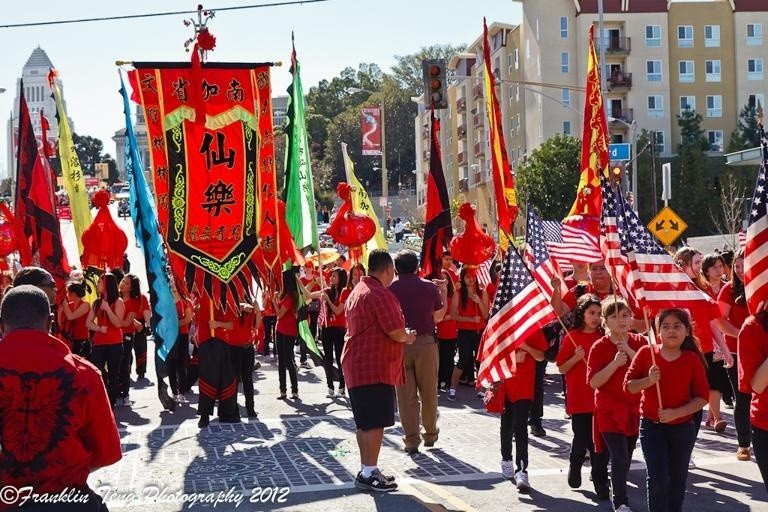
[198,415,209,428]
[737,446,751,461]
[501,458,515,478]
[514,469,531,491]
[592,472,632,512]
[530,423,546,436]
[262,352,313,369]
[704,416,728,432]
[424,436,439,447]
[437,378,485,402]
[116,377,154,407]
[219,415,241,423]
[568,462,581,489]
[688,458,695,468]
[277,394,297,399]
[403,445,419,453]
[354,467,398,492]
[327,387,345,398]
[171,388,199,404]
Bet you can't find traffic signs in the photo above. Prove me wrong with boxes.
[609,143,630,161]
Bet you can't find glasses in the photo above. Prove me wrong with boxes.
[35,281,56,289]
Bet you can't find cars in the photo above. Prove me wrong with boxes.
[316,220,423,274]
[103,180,130,220]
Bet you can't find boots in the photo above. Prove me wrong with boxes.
[245,401,257,416]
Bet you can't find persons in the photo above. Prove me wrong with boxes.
[1,238,768,512]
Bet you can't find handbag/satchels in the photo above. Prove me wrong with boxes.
[306,302,320,312]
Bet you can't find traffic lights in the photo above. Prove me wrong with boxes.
[422,58,449,111]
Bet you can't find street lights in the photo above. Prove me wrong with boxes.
[6,107,40,211]
[347,87,390,243]
[274,110,286,116]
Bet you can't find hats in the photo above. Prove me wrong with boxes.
[305,260,313,268]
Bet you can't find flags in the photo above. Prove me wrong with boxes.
[12,13,768,414]
